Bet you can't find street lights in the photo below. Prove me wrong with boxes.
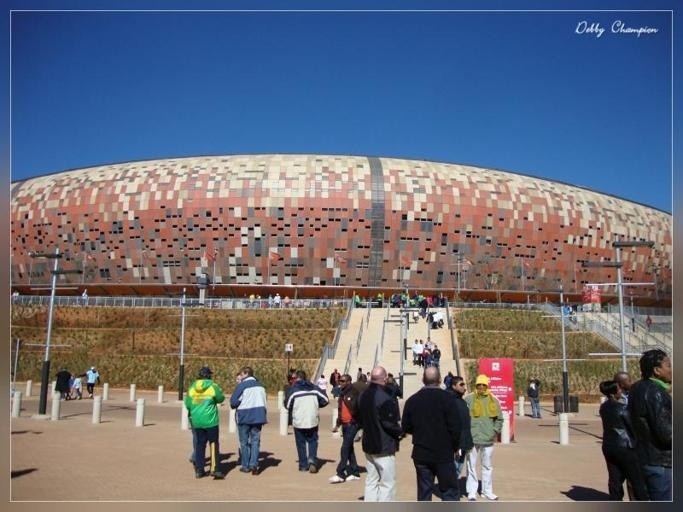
[535,281,574,412]
[164,286,203,399]
[26,245,84,413]
[579,233,655,380]
[385,283,422,395]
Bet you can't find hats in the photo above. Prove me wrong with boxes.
[199,367,213,376]
[475,374,489,388]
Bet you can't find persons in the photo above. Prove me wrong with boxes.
[352,290,451,330]
[53,366,101,401]
[646,316,652,333]
[528,377,541,418]
[12,290,19,301]
[244,293,300,311]
[83,289,87,305]
[600,349,671,501]
[561,301,583,324]
[183,337,504,505]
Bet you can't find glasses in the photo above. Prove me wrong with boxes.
[459,383,465,387]
[339,380,345,383]
[478,384,486,386]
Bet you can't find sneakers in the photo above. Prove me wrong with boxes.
[190,458,361,483]
[481,493,499,500]
[467,493,478,501]
